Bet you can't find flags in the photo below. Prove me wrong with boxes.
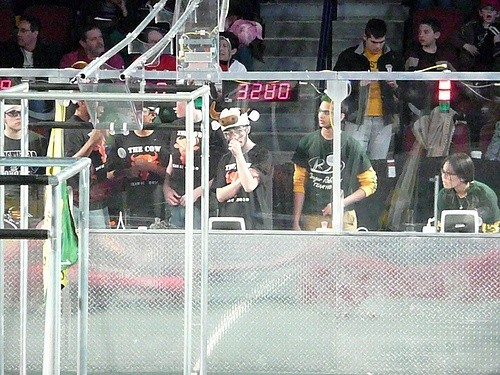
[43,90,79,295]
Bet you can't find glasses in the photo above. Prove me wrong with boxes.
[440,168,454,176]
[15,27,31,30]
[6,110,22,114]
[371,38,386,45]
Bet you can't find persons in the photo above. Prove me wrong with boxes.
[209,101,273,230]
[437,152,500,233]
[332,18,411,161]
[455,0,500,101]
[0,0,264,230]
[292,95,378,230]
[403,15,459,125]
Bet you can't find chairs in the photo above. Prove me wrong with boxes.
[0,5,72,47]
[406,9,495,157]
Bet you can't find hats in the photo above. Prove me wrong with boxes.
[211,109,259,132]
[479,0,500,12]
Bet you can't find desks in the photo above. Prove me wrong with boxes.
[3,232,500,310]
